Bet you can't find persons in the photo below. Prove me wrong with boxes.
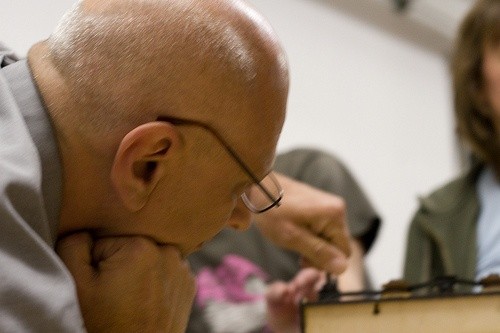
[189,148,380,333]
[402,0,500,290]
[0,0,352,333]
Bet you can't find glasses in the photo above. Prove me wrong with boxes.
[156,114,283,214]
